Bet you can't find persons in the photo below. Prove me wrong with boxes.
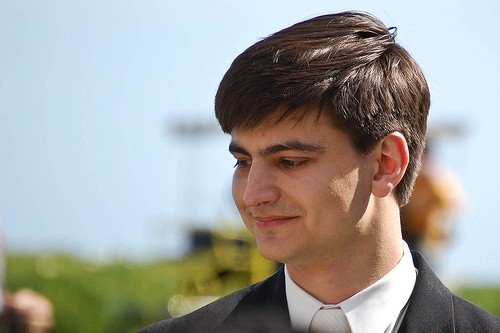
[399,132,453,275]
[136,11,500,333]
